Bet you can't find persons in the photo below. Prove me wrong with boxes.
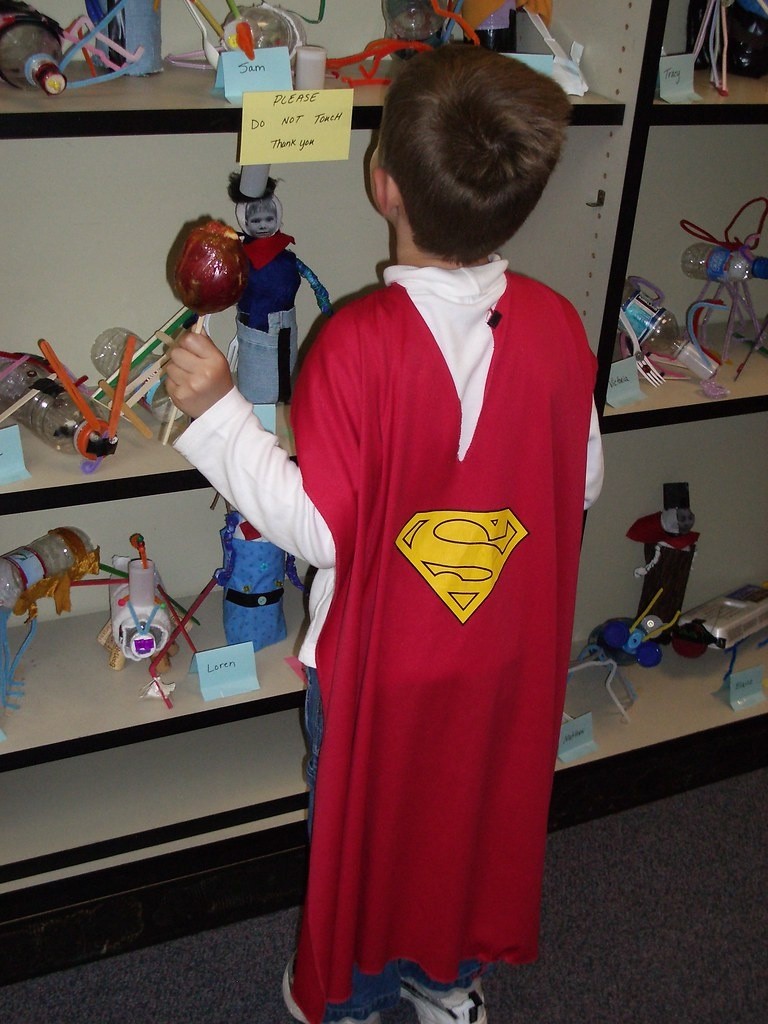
[156,43,608,1022]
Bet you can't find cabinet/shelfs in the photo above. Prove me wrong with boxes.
[0,0,767,988]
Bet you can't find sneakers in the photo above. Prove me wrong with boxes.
[398,975,488,1024]
[280,946,381,1024]
